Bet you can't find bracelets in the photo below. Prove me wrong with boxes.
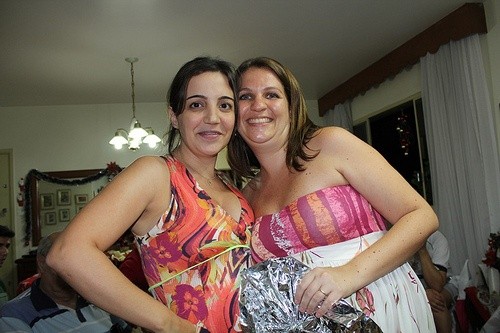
[195,326,211,333]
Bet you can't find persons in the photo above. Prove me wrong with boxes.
[0,226,134,333]
[406,229,458,333]
[225,57,439,333]
[46,55,254,333]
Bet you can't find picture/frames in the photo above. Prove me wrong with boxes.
[40,188,89,226]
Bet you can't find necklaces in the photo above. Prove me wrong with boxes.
[179,147,220,189]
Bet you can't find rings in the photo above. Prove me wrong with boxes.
[317,289,328,296]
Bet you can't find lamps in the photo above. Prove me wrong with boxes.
[109,56,162,151]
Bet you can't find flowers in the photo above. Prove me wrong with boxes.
[482,232,500,272]
